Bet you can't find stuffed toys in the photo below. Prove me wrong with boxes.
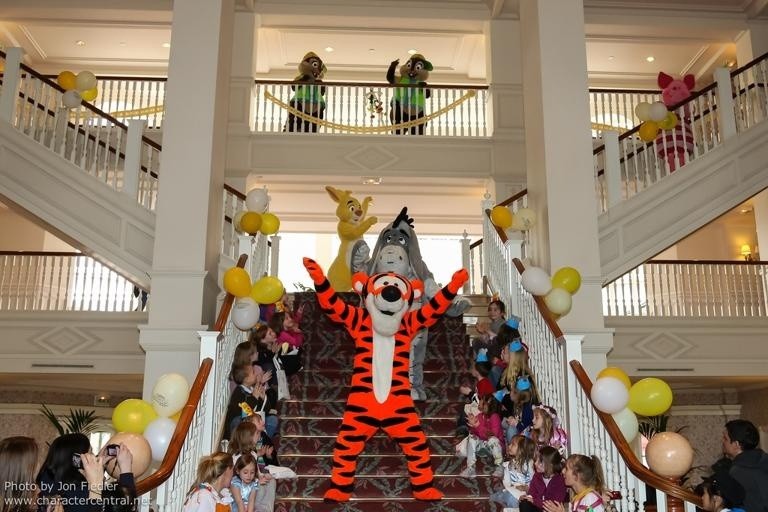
[387,53,433,136]
[325,185,376,292]
[304,257,470,504]
[350,206,472,401]
[283,50,327,135]
[657,71,696,170]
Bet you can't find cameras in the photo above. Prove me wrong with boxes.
[71,452,83,470]
[107,444,120,456]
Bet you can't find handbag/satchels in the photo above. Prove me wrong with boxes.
[253,395,267,424]
[256,464,297,480]
[273,357,291,401]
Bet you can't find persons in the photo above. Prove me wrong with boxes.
[227,422,276,511]
[699,474,746,512]
[252,325,302,383]
[232,454,259,512]
[226,364,278,437]
[244,414,279,467]
[230,340,269,396]
[455,301,606,512]
[36,433,135,512]
[0,436,107,512]
[266,288,304,322]
[182,452,232,511]
[271,313,304,348]
[722,419,768,512]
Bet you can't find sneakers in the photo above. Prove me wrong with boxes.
[461,467,476,478]
[492,465,505,477]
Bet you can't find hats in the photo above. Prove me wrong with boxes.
[241,401,252,419]
[492,387,507,402]
[516,374,530,390]
[476,348,488,362]
[509,337,522,351]
[506,314,521,329]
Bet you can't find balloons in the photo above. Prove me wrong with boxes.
[596,366,632,389]
[62,91,81,107]
[232,298,260,330]
[491,206,511,228]
[628,378,672,415]
[261,211,279,235]
[77,71,95,89]
[58,71,76,89]
[102,433,150,480]
[614,407,638,442]
[658,110,677,129]
[241,212,261,234]
[552,266,582,292]
[224,267,251,297]
[82,90,98,100]
[635,102,651,121]
[591,378,628,414]
[646,432,692,477]
[246,189,268,214]
[522,268,551,296]
[153,374,188,418]
[650,103,666,120]
[252,277,284,304]
[113,398,153,434]
[544,290,571,314]
[512,209,535,230]
[143,418,176,460]
[640,123,657,141]
